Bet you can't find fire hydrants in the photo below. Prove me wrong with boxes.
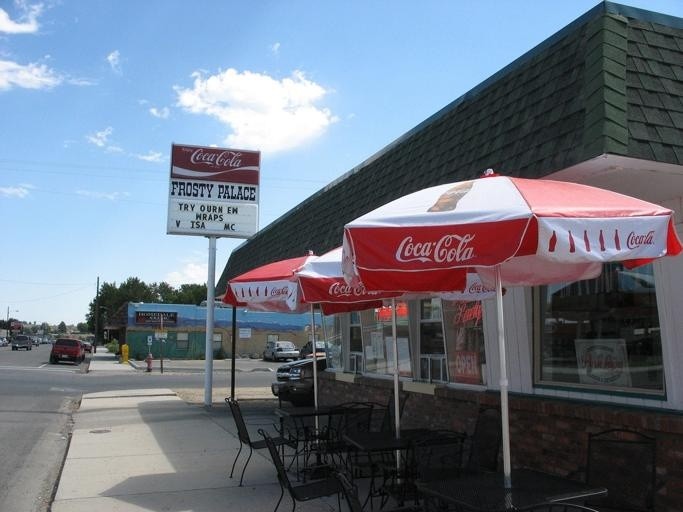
[145,352,153,372]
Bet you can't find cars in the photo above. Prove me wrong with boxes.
[0,337,10,347]
[50,338,92,365]
[262,341,333,407]
[12,335,48,350]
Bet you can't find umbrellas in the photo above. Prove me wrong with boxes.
[291,240,510,482]
[340,163,680,491]
[221,252,401,449]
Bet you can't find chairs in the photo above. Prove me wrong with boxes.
[335,474,422,512]
[546,428,656,512]
[225,397,296,489]
[257,428,343,512]
[278,392,409,484]
[362,404,502,512]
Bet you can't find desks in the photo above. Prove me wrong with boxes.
[341,428,466,512]
[414,467,610,512]
[272,405,384,473]
